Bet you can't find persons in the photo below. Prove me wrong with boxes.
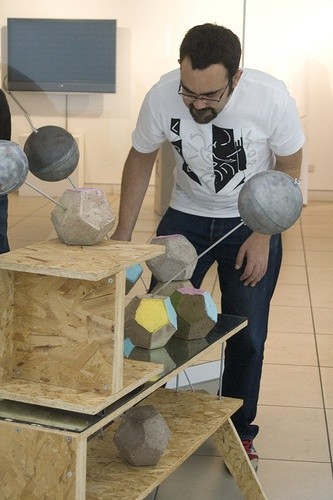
[110,22,307,478]
[0,89,12,253]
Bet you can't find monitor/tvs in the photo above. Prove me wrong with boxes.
[7,18,117,93]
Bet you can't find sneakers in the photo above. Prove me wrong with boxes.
[224,440,258,476]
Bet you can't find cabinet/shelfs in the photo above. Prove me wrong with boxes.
[1,233,268,500]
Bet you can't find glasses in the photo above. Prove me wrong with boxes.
[178,82,228,102]
